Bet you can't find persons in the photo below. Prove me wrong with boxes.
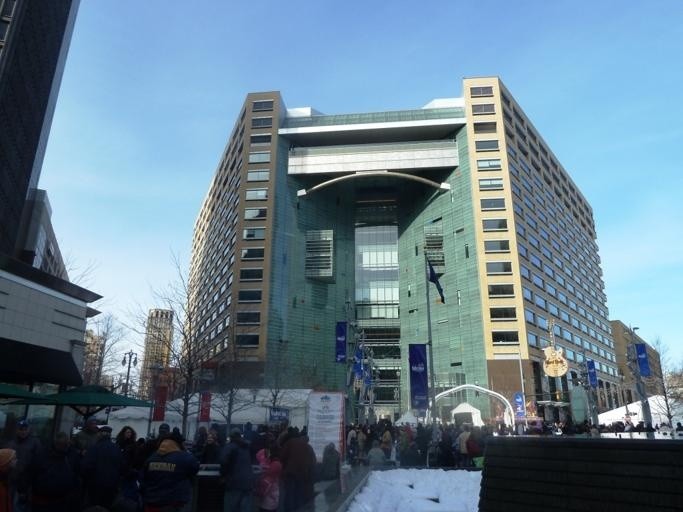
[483,416,682,437]
[1,417,318,512]
[324,442,340,479]
[346,419,489,470]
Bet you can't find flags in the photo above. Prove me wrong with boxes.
[426,257,446,304]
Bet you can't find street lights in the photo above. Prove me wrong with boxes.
[395,368,401,422]
[578,347,600,428]
[617,369,631,420]
[349,321,377,423]
[145,356,165,440]
[623,323,654,431]
[121,349,138,398]
